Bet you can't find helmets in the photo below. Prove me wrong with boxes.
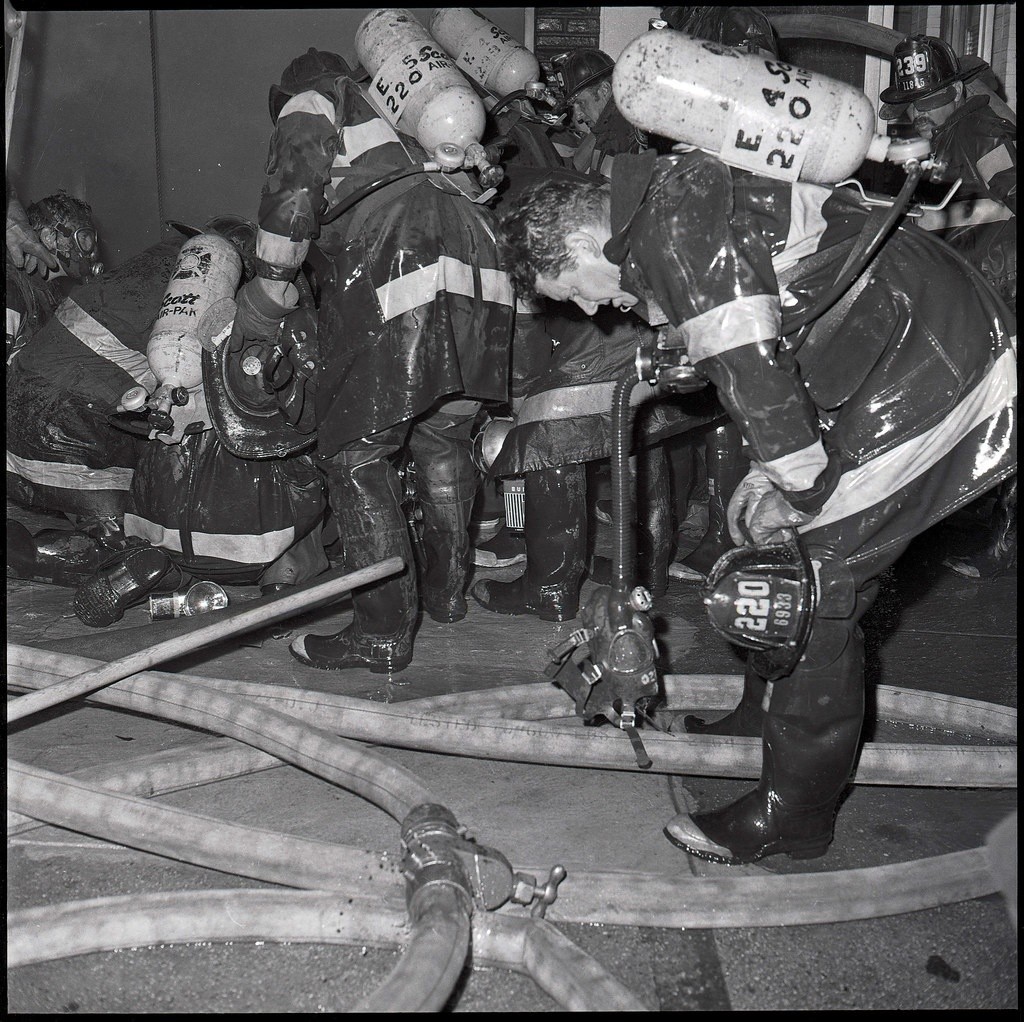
[549,48,615,101]
[168,214,262,280]
[701,535,815,681]
[879,34,959,107]
[269,45,350,124]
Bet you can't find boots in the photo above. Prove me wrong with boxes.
[289,412,417,674]
[471,461,589,620]
[5,515,123,588]
[662,676,867,865]
[682,648,780,732]
[669,421,745,584]
[942,475,1023,580]
[74,545,185,628]
[589,440,674,596]
[401,411,480,623]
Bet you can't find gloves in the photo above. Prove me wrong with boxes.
[225,279,298,353]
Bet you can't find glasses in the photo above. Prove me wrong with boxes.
[72,226,98,256]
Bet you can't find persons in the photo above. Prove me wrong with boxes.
[491,149,1020,865]
[6,46,748,676]
[879,33,1019,578]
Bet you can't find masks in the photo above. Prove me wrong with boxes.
[56,252,104,286]
